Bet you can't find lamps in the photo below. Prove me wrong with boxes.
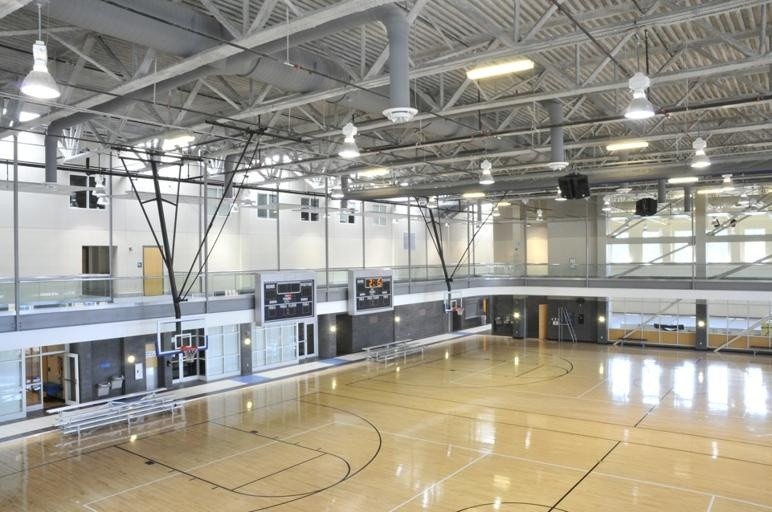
[687,135,712,169]
[478,161,497,185]
[623,72,659,121]
[336,117,360,159]
[720,174,736,191]
[14,4,66,102]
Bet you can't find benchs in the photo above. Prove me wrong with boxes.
[616,337,649,350]
[360,337,430,369]
[42,382,180,444]
[744,344,772,360]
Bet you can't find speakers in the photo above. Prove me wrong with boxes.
[635,198,656,215]
[558,174,590,198]
